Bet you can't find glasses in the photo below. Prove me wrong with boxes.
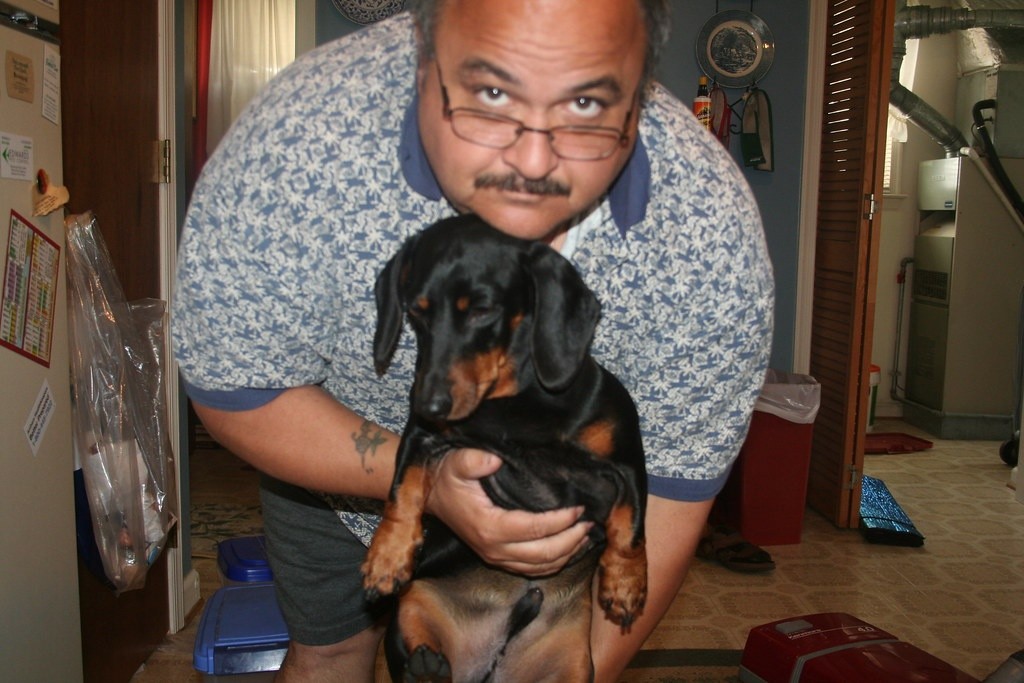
[432,35,646,161]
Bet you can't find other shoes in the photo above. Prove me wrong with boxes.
[696,536,775,573]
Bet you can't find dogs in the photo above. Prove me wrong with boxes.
[358,212,647,683]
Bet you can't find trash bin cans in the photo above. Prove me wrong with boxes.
[739,370,821,546]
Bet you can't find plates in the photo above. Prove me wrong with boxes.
[695,9,775,89]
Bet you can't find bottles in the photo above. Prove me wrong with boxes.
[693,76,711,131]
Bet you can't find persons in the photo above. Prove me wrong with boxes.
[170,0,775,683]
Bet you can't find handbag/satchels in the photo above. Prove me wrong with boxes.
[64,210,176,593]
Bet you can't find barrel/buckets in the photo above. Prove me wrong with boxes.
[865,364,881,434]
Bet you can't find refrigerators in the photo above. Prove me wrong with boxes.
[0,0,84,683]
